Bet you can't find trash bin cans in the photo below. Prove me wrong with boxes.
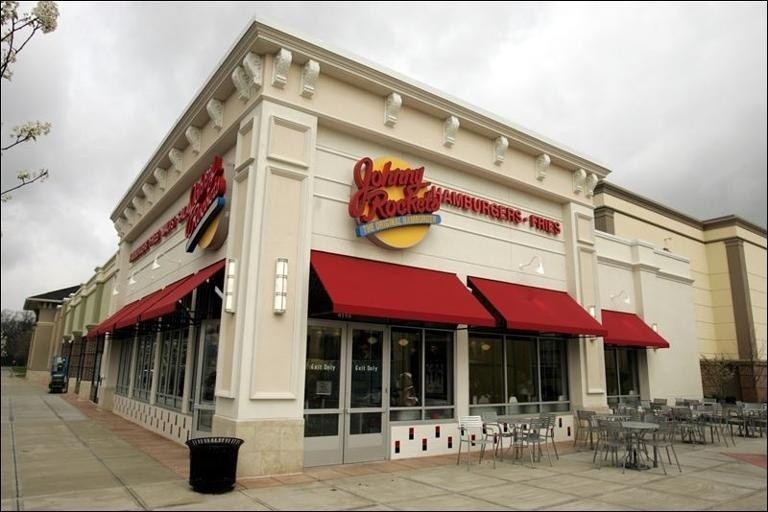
[185,437,244,493]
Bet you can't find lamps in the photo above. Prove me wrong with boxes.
[611,288,632,304]
[151,251,182,272]
[128,270,154,286]
[273,257,289,315]
[223,256,238,316]
[588,305,597,343]
[519,255,545,277]
[112,282,132,297]
